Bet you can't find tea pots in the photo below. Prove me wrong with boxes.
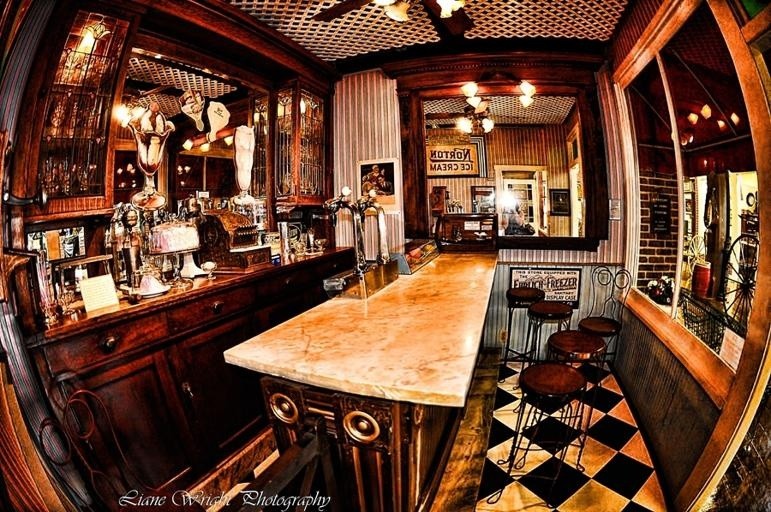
[395,70,612,253]
[111,43,269,218]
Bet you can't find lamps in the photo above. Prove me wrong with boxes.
[567,266,633,392]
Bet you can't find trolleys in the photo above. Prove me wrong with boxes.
[311,1,477,33]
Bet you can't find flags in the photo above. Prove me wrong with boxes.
[652,294,674,306]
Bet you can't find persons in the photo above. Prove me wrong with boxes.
[503,200,525,235]
[362,165,392,194]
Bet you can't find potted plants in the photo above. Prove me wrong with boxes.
[508,267,582,309]
[550,187,571,216]
[471,185,497,214]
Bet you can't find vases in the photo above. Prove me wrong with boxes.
[646,274,676,298]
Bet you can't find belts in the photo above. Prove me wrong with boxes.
[299,232,308,256]
[307,229,316,250]
[314,239,326,250]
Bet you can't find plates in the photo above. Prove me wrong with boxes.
[38,307,210,507]
[21,3,132,224]
[164,275,276,466]
[440,212,499,241]
[320,245,357,304]
[273,80,332,216]
[260,256,320,334]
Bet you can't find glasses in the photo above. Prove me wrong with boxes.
[464,96,534,108]
[678,129,694,148]
[717,111,740,132]
[116,88,150,128]
[373,1,468,24]
[460,69,538,99]
[685,104,712,126]
[458,104,496,134]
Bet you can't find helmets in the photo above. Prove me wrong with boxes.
[132,285,171,300]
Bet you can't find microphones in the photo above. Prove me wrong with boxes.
[516,331,608,472]
[500,288,547,388]
[511,300,574,414]
[486,363,586,510]
